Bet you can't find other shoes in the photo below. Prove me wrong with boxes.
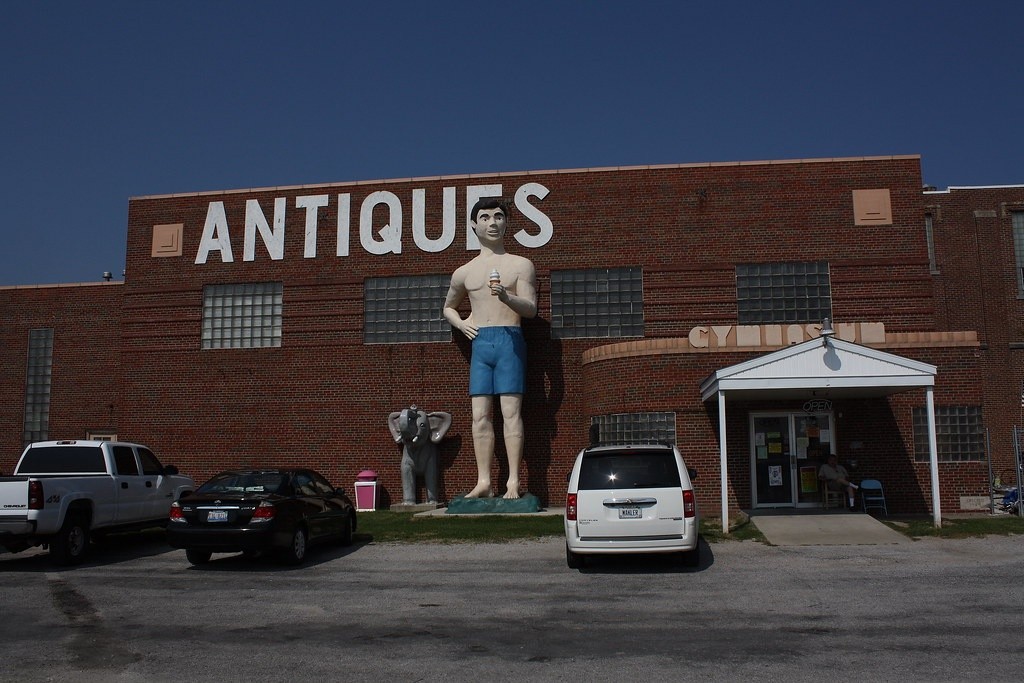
[857,485,863,492]
[850,507,856,512]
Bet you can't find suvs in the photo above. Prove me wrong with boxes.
[564,442,700,567]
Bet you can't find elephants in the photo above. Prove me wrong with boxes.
[388,408,452,505]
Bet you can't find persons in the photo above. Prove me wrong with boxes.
[443,198,537,499]
[818,454,862,512]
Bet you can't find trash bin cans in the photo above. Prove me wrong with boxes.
[353,469,382,512]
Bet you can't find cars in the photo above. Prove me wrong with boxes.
[169,465,356,567]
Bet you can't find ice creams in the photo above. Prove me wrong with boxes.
[489,267,500,295]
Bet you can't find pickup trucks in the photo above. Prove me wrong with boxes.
[0,440,195,565]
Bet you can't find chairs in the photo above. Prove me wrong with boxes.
[822,481,847,511]
[860,479,888,516]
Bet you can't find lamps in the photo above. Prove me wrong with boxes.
[819,318,837,349]
